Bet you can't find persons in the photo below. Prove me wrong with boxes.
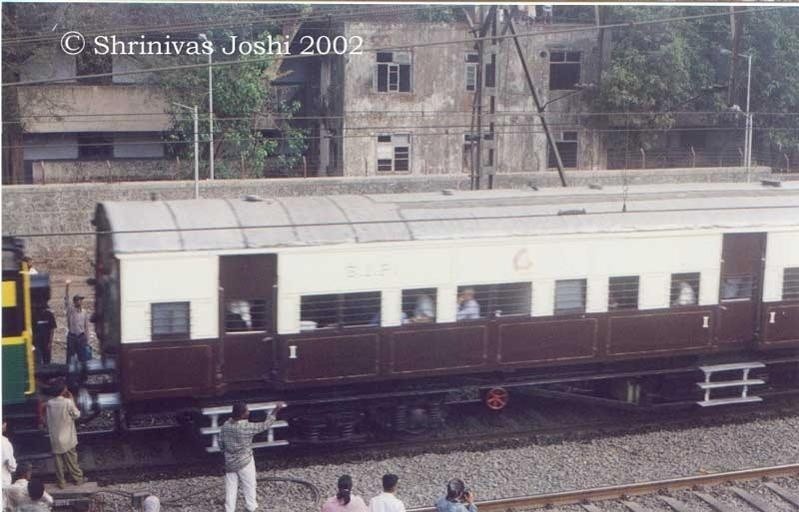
[216,397,283,511]
[371,286,481,326]
[18,256,38,274]
[38,378,88,488]
[64,278,90,373]
[435,477,478,512]
[324,475,367,511]
[2,413,19,493]
[5,460,54,511]
[14,480,51,512]
[364,473,407,512]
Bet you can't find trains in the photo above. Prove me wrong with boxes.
[3,161,798,448]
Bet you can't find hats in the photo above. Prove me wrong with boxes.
[73,294,84,301]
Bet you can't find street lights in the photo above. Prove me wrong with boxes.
[715,48,752,169]
[727,103,754,170]
[198,33,218,181]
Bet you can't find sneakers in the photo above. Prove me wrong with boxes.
[74,475,89,486]
[56,480,68,489]
